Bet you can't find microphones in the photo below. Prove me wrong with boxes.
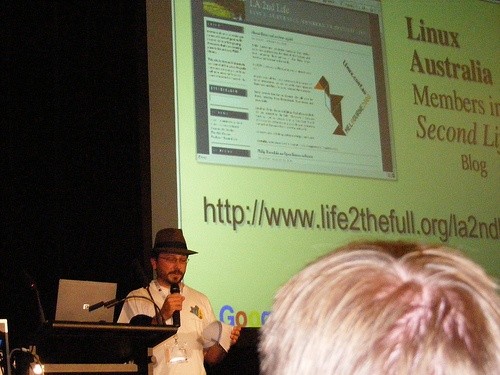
[141,280,162,325]
[105,293,166,326]
[26,279,49,326]
[170,282,180,327]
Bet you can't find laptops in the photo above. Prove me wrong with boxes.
[54,278,118,325]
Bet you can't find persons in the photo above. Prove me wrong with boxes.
[116,227,243,375]
[256,239,500,375]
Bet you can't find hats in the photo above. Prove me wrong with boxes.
[148,228,198,257]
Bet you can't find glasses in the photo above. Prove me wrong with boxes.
[155,254,190,265]
